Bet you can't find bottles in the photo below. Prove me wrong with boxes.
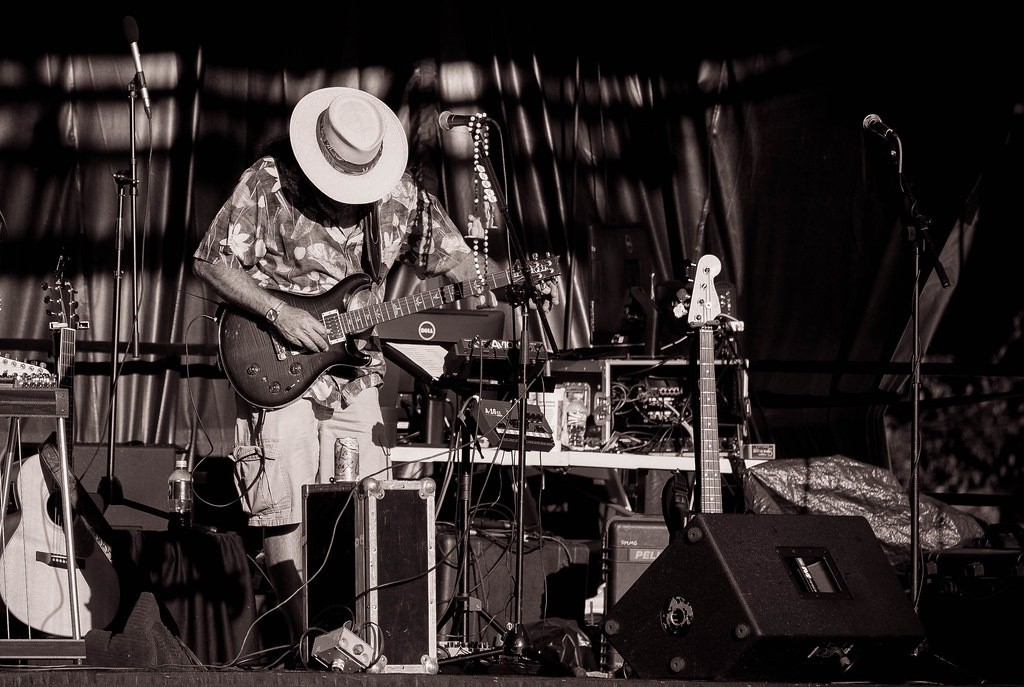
[566,393,588,451]
[167,461,194,530]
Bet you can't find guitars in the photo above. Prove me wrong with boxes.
[213,253,562,412]
[686,253,726,515]
[1,254,124,644]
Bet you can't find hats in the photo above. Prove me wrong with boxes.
[289,86,409,205]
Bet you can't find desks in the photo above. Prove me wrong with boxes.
[391,446,768,474]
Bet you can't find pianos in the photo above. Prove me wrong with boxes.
[378,296,506,348]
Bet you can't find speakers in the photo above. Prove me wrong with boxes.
[73,443,186,531]
[601,512,919,683]
[894,549,1024,687]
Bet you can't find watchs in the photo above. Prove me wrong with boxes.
[264,300,287,323]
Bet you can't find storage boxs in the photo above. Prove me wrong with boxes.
[302,477,439,675]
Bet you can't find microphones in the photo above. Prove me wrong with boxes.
[120,16,151,121]
[862,114,900,140]
[438,110,495,131]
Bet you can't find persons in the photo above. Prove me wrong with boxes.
[195,88,561,644]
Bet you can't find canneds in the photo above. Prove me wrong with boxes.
[333,437,360,482]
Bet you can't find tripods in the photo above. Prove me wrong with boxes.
[436,128,585,682]
[95,79,218,539]
